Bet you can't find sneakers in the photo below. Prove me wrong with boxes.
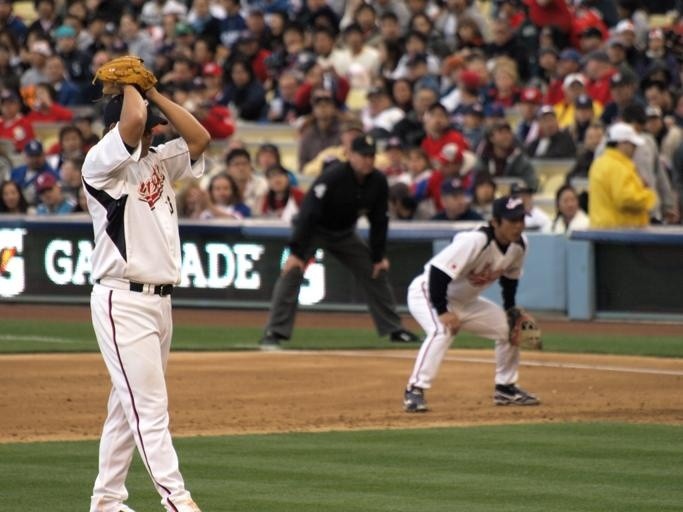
[387,330,419,343]
[402,385,429,413]
[256,331,282,352]
[492,384,542,408]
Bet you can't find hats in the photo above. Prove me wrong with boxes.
[605,120,645,147]
[536,105,556,120]
[103,93,168,131]
[388,182,411,200]
[493,195,533,220]
[351,133,377,155]
[558,20,677,108]
[36,173,57,193]
[24,139,41,156]
[511,181,536,194]
[0,88,18,104]
[435,142,463,166]
[440,175,468,196]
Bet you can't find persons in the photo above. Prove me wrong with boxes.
[403,193,542,414]
[77,55,215,512]
[256,135,421,352]
[0,1,683,231]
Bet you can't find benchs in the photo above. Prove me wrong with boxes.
[0,0,682,222]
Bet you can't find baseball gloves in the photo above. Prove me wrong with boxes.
[92,56,157,96]
[505,304,542,350]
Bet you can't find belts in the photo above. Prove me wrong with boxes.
[92,278,176,297]
